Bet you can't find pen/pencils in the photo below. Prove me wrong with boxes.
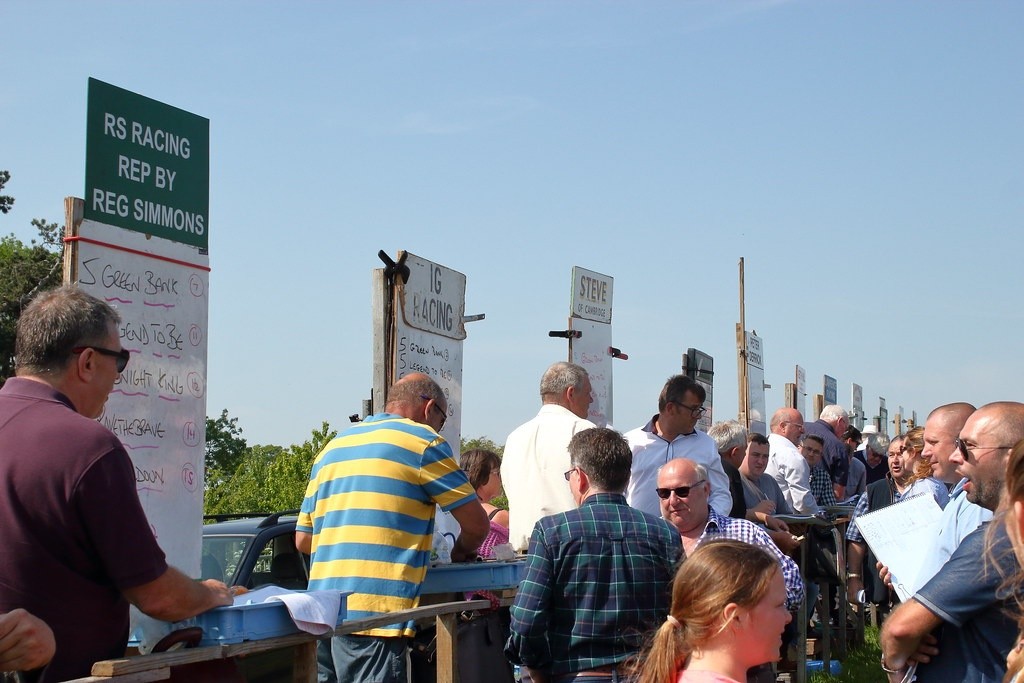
[797,535,805,541]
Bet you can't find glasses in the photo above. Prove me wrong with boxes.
[420,394,447,432]
[668,400,707,418]
[868,446,884,459]
[839,416,850,430]
[954,438,1013,461]
[564,468,588,481]
[900,445,911,454]
[800,444,821,457]
[70,345,130,373]
[656,480,706,499]
[782,421,803,431]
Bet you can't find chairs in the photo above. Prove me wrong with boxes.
[271,553,306,590]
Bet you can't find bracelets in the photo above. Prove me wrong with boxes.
[849,573,859,578]
[881,654,905,675]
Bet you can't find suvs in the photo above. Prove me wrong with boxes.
[200,509,312,596]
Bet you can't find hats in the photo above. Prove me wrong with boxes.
[858,425,877,434]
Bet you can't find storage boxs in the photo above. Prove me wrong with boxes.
[422,561,525,596]
[168,589,356,647]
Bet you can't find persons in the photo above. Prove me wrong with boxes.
[624,540,792,683]
[707,404,949,638]
[296,371,490,683]
[622,375,732,517]
[883,402,1024,683]
[459,450,509,611]
[500,361,597,557]
[657,458,805,683]
[0,287,233,683]
[504,428,687,683]
